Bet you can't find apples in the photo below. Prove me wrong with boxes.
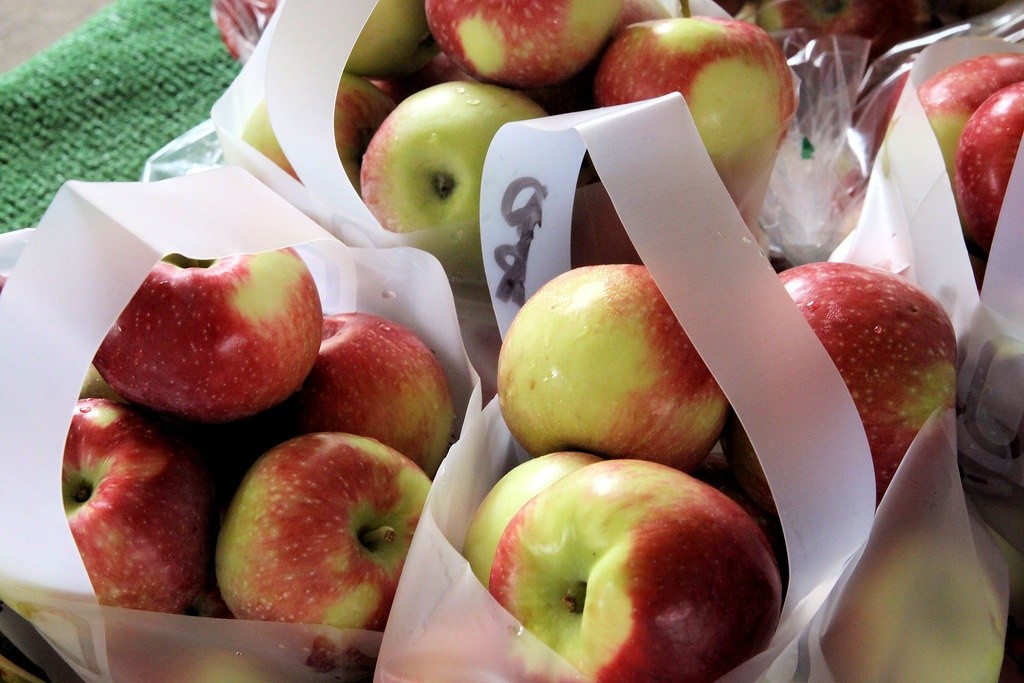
[59,0,1024,683]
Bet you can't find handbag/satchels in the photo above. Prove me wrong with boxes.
[2,1,1023,682]
